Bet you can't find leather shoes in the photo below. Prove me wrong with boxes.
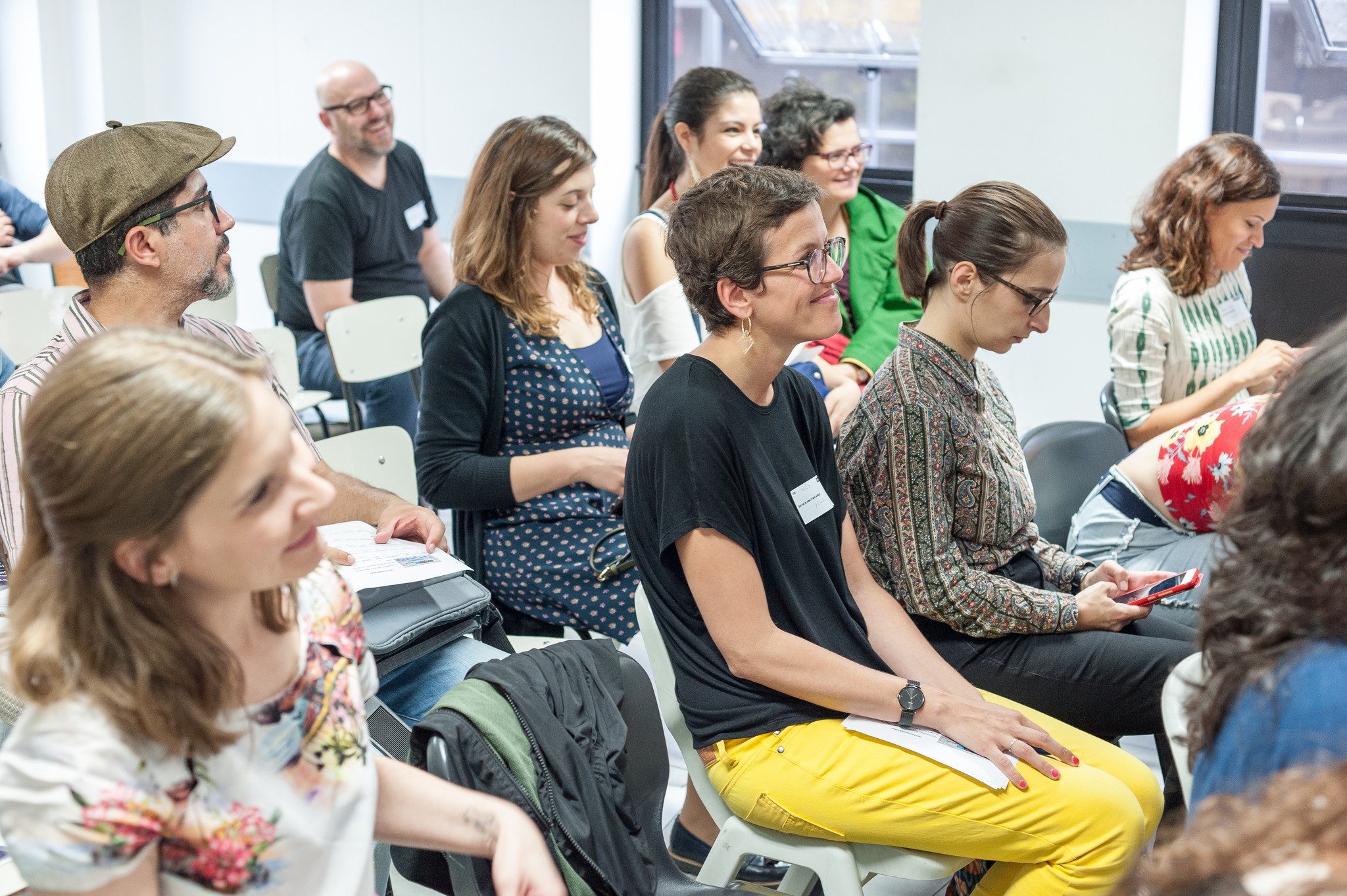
[666,815,792,890]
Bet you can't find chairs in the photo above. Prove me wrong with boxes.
[0,256,1214,895]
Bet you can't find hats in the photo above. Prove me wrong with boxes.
[43,121,236,253]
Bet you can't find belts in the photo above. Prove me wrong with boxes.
[1099,471,1170,528]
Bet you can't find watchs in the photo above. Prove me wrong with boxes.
[896,680,925,727]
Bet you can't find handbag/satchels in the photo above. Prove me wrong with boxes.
[946,855,994,896]
[356,554,490,677]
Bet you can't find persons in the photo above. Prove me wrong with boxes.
[1065,393,1286,642]
[623,164,1162,896]
[0,174,75,288]
[1106,132,1317,449]
[623,65,859,436]
[277,57,460,442]
[834,182,1204,811]
[415,114,645,643]
[0,123,516,728]
[1180,314,1347,815]
[1136,757,1347,895]
[0,328,566,895]
[760,79,925,387]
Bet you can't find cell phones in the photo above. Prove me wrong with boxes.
[1112,567,1201,607]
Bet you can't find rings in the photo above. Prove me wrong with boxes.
[1008,739,1018,752]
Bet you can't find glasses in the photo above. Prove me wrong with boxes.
[948,267,1059,317]
[325,85,393,115]
[811,140,875,169]
[118,190,221,257]
[762,236,846,285]
